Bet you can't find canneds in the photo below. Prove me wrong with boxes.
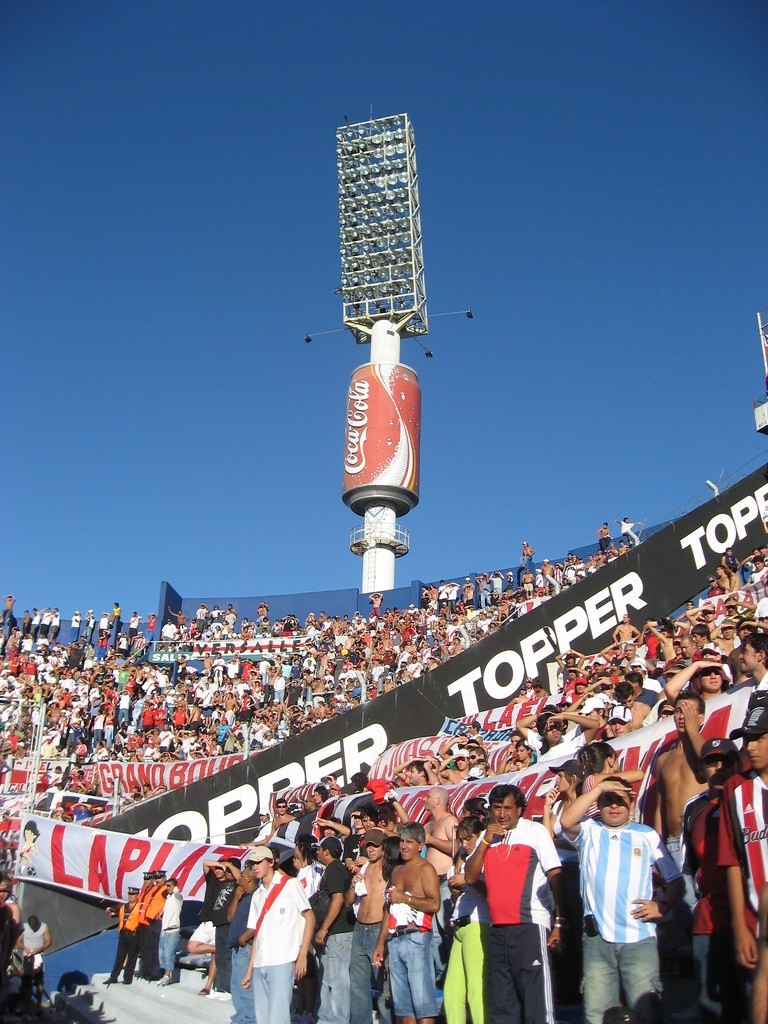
[342,361,421,517]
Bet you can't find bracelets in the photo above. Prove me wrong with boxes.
[482,839,489,844]
[320,927,327,931]
[555,916,568,928]
[409,897,411,902]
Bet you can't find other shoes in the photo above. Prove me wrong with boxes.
[161,975,175,984]
[123,980,131,984]
[103,978,118,984]
[291,1010,315,1024]
[197,989,210,994]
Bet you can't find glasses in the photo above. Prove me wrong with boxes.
[277,806,287,809]
[467,756,476,760]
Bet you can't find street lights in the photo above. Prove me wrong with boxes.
[303,112,473,595]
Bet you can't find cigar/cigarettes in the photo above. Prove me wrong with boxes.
[372,962,379,965]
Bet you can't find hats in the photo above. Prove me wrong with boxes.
[548,760,586,782]
[152,870,167,878]
[245,846,273,863]
[365,829,386,846]
[311,837,343,856]
[701,738,740,765]
[517,575,768,740]
[259,808,269,816]
[143,872,153,880]
[127,887,139,894]
[456,749,469,758]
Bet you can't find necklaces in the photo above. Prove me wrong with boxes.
[496,843,511,862]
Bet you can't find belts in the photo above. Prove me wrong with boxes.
[394,929,421,937]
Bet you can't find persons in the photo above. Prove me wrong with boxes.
[521,542,535,568]
[0,541,768,1024]
[615,518,642,546]
[597,522,611,551]
